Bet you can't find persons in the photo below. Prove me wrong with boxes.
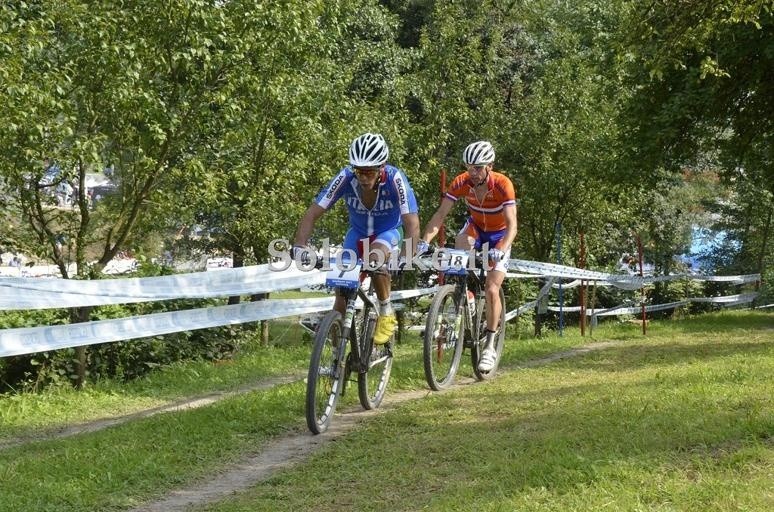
[414,139,519,375]
[289,133,420,397]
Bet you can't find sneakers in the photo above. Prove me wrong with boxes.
[466,299,477,315]
[373,310,398,344]
[478,345,497,373]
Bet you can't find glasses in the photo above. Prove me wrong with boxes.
[468,166,488,172]
[355,167,381,179]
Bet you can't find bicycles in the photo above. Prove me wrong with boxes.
[417,245,506,391]
[290,249,408,434]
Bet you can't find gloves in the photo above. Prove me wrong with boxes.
[389,256,406,271]
[487,247,505,261]
[417,240,429,252]
[293,246,308,261]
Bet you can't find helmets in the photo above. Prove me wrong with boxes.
[463,141,496,167]
[348,132,390,167]
